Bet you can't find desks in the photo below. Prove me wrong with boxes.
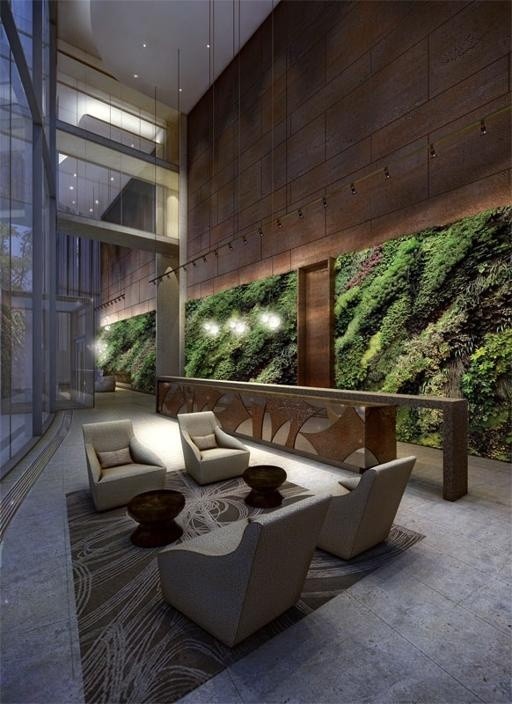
[242,465,287,508]
[128,490,185,547]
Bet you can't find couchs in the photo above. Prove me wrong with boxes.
[158,493,334,648]
[81,418,166,511]
[282,456,417,561]
[176,410,250,485]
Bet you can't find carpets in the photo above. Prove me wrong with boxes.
[63,468,426,703]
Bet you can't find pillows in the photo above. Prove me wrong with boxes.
[191,433,218,451]
[97,446,133,469]
[338,475,361,491]
